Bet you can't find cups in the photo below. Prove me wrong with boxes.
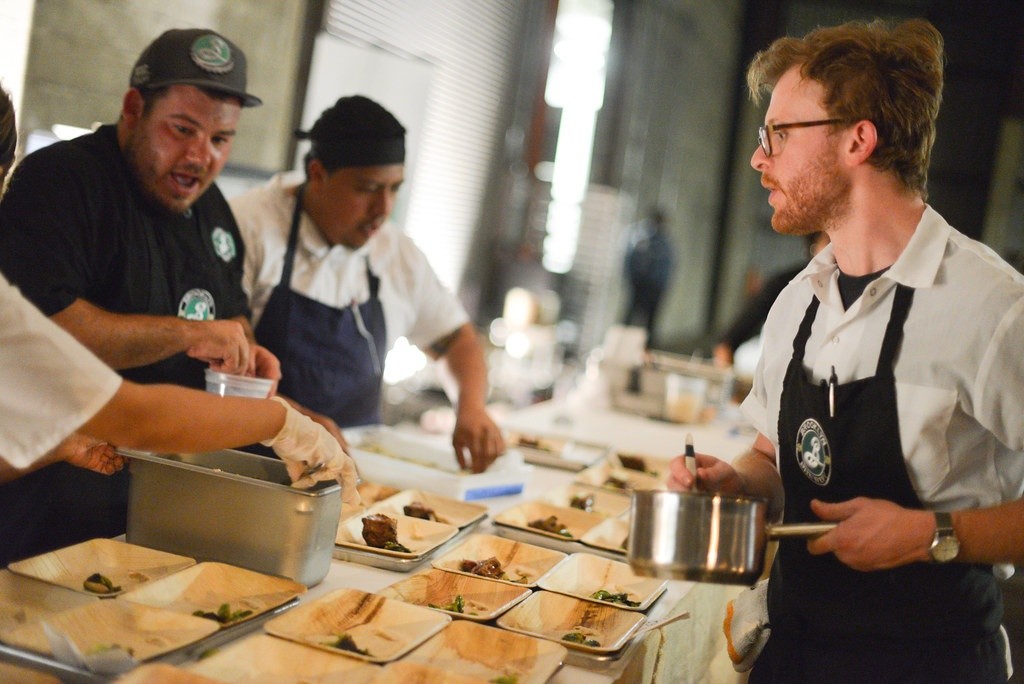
[202,367,274,404]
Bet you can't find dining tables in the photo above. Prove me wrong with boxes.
[1,384,776,683]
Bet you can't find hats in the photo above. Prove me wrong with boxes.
[129,27,261,107]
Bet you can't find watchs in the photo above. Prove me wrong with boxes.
[932,509,960,567]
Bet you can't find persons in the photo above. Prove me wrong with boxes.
[3,91,362,493]
[1,25,352,460]
[621,204,678,345]
[708,230,831,355]
[224,94,510,478]
[665,16,1023,682]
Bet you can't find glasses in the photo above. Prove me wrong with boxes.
[756,117,849,157]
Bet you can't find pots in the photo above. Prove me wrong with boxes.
[626,487,840,586]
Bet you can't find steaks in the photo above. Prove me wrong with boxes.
[362,514,397,547]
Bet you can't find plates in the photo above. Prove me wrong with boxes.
[0,429,701,684]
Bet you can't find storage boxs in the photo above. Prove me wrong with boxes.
[115,446,361,589]
[600,350,735,423]
